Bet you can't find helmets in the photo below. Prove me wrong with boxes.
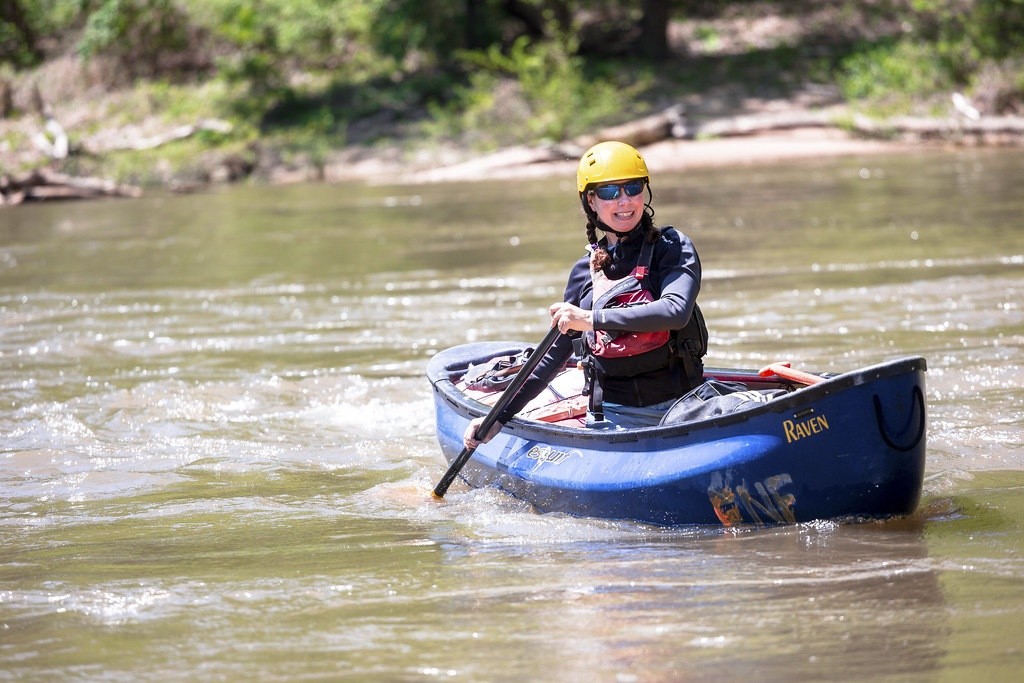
[577,141,650,200]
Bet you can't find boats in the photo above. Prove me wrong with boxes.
[427,339,927,529]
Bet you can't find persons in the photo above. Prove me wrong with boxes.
[462,141,708,452]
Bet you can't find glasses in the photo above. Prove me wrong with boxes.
[588,179,646,200]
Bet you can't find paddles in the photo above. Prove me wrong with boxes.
[428,321,565,500]
[757,360,829,386]
